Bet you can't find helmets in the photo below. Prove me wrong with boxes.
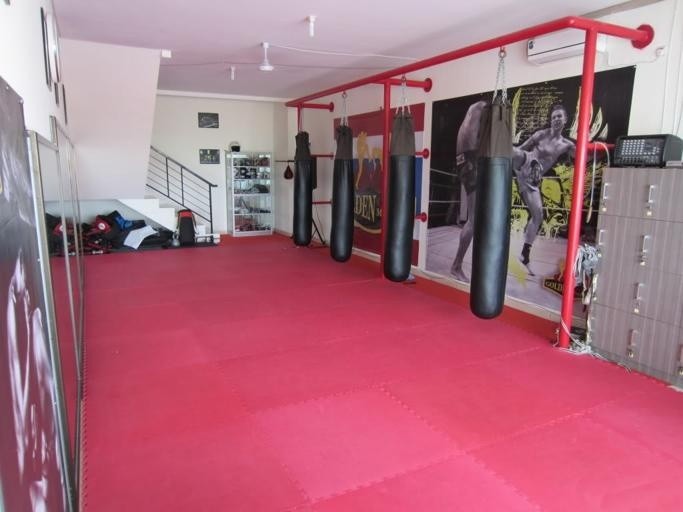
[228,141,240,152]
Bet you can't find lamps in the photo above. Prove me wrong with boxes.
[307,15,316,37]
[258,42,273,72]
[229,66,236,80]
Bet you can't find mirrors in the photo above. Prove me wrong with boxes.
[30,115,86,512]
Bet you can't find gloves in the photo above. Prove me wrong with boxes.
[460,160,479,190]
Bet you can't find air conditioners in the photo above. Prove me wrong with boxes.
[527,22,605,66]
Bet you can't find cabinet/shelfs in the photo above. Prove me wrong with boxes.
[587,168,683,391]
[225,150,273,238]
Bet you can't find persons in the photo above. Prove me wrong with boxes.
[449,100,489,283]
[509,106,575,277]
